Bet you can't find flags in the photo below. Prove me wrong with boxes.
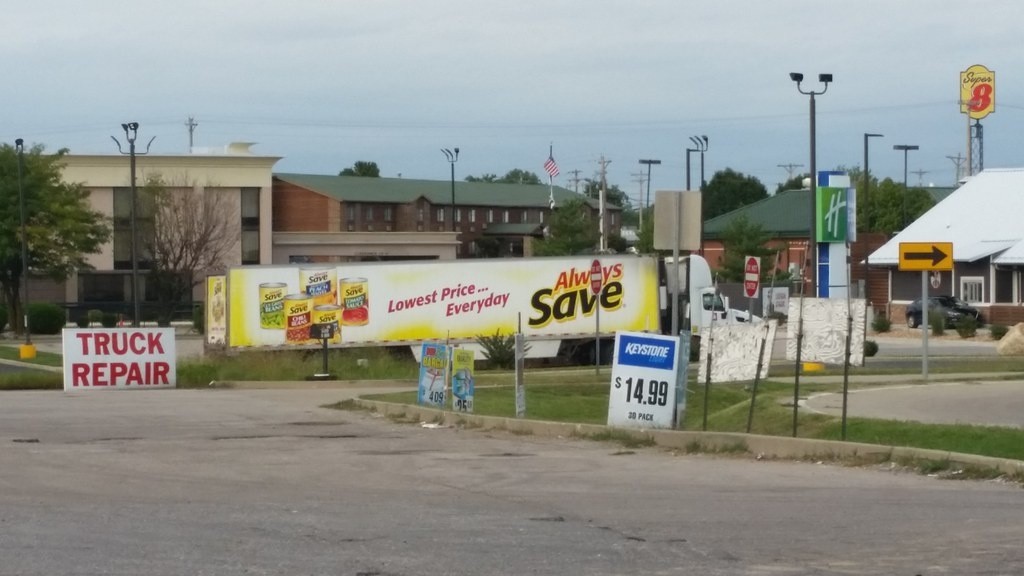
[544,157,560,178]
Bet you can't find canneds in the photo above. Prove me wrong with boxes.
[259,266,369,345]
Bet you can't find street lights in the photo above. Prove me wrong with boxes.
[441,145,462,231]
[790,72,833,296]
[860,131,883,304]
[111,122,158,326]
[638,159,661,206]
[892,143,919,225]
[16,137,35,359]
[689,133,710,190]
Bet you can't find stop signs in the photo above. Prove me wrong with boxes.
[743,256,764,300]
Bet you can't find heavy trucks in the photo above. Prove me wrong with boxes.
[203,252,747,373]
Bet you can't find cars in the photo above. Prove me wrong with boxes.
[906,296,980,329]
